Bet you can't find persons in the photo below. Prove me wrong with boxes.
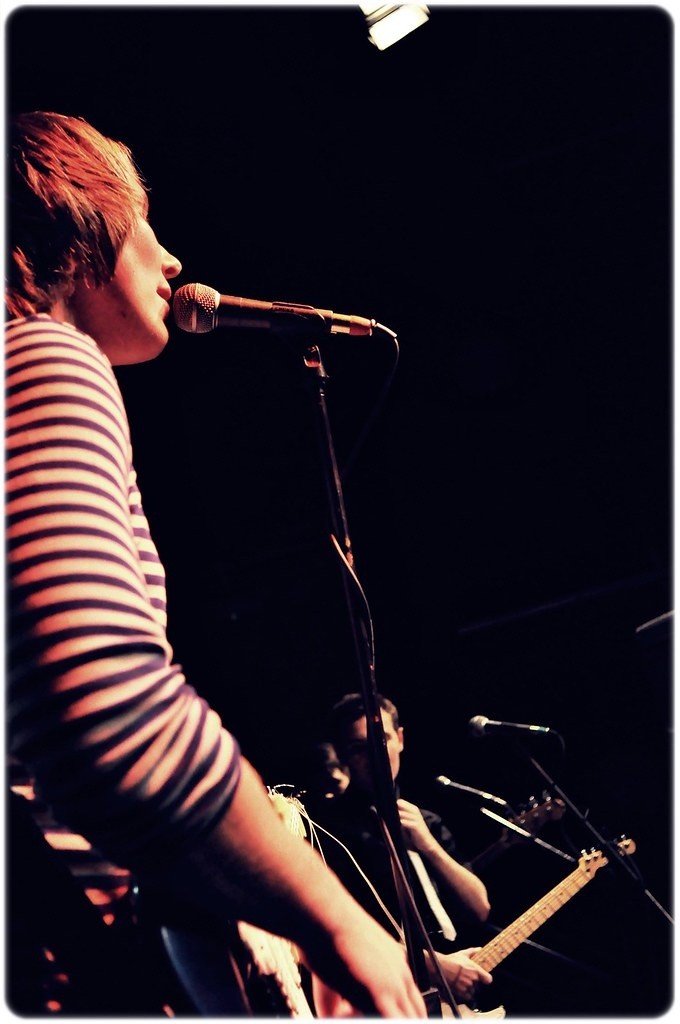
[308,691,493,1001]
[4,111,427,1018]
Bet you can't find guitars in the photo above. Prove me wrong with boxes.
[459,833,640,971]
[383,787,564,951]
[6,785,511,1021]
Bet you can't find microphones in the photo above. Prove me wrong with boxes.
[170,280,379,340]
[434,774,507,809]
[469,715,550,740]
